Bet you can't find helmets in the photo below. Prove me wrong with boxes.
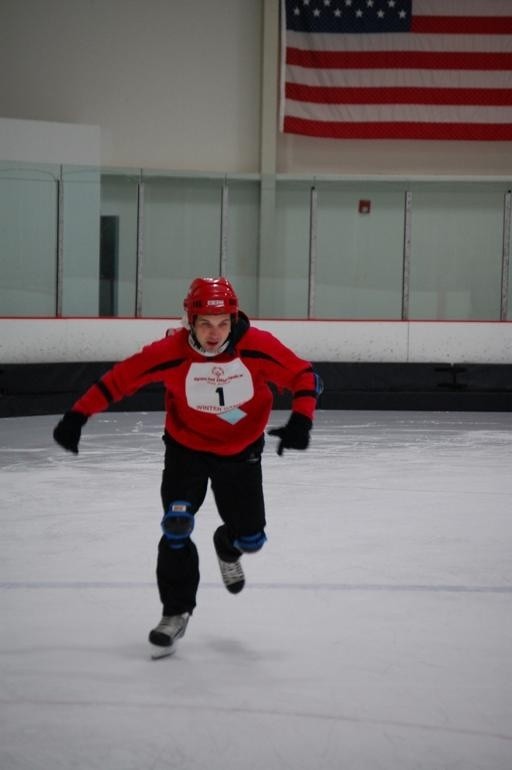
[183,276,239,324]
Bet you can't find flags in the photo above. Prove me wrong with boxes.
[278,0,511,146]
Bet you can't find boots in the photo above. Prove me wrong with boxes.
[149,609,191,646]
[218,556,245,594]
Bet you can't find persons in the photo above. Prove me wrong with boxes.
[51,277,327,648]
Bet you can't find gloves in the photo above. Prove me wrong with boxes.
[267,411,314,456]
[52,408,88,457]
